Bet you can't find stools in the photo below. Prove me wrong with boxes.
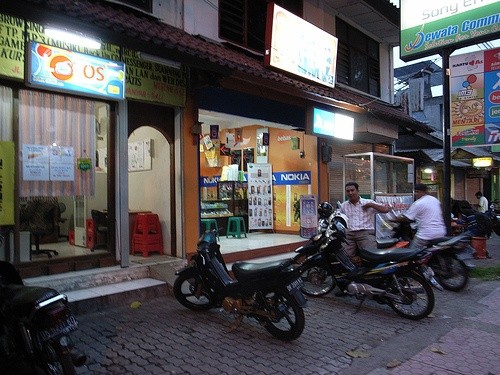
[201,219,220,241]
[132,212,163,258]
[226,217,247,239]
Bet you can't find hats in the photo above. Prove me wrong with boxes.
[415,183,426,192]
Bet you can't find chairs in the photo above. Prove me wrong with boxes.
[91,209,110,252]
[24,201,60,258]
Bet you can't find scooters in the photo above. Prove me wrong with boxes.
[488,198,500,237]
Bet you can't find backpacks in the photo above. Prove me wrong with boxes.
[464,209,493,234]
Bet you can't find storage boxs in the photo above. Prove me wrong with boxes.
[86,219,95,249]
[69,230,74,245]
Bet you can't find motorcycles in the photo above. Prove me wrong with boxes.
[292,200,444,320]
[173,226,325,341]
[0,260,88,375]
[387,217,477,292]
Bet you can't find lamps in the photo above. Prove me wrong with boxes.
[137,52,182,70]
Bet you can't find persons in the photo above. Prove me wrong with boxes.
[457,208,491,258]
[335,182,389,297]
[452,191,488,217]
[391,183,447,252]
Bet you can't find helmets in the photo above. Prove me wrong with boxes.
[329,210,349,229]
[317,202,332,216]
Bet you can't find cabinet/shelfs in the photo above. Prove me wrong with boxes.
[201,181,248,237]
